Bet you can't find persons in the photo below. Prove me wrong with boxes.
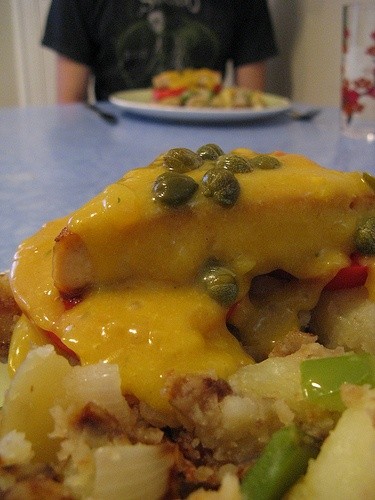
[40,1,279,107]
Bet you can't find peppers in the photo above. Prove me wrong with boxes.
[299,354,374,410]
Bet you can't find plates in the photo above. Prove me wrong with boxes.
[107,88,293,122]
